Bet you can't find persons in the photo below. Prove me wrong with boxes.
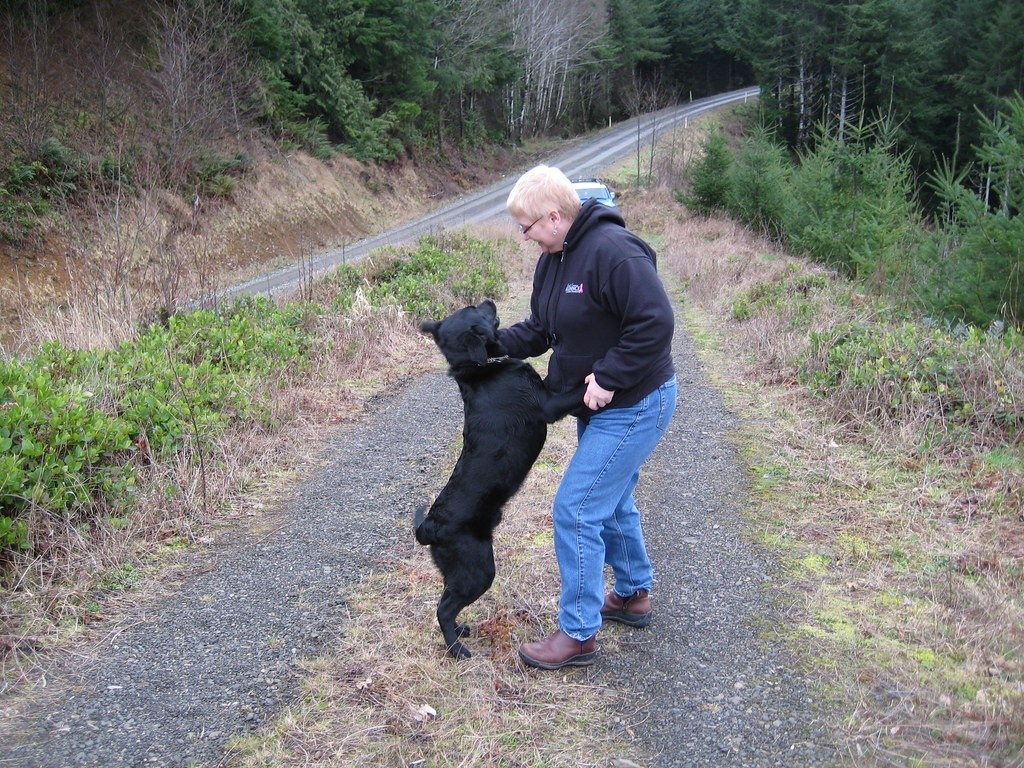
[485,163,677,670]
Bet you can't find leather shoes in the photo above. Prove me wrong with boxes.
[600,588,653,627]
[518,628,596,669]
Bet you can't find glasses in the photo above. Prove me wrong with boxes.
[520,212,548,238]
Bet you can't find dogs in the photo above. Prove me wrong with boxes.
[413,298,590,660]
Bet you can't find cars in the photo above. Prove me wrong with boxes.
[571,178,617,211]
